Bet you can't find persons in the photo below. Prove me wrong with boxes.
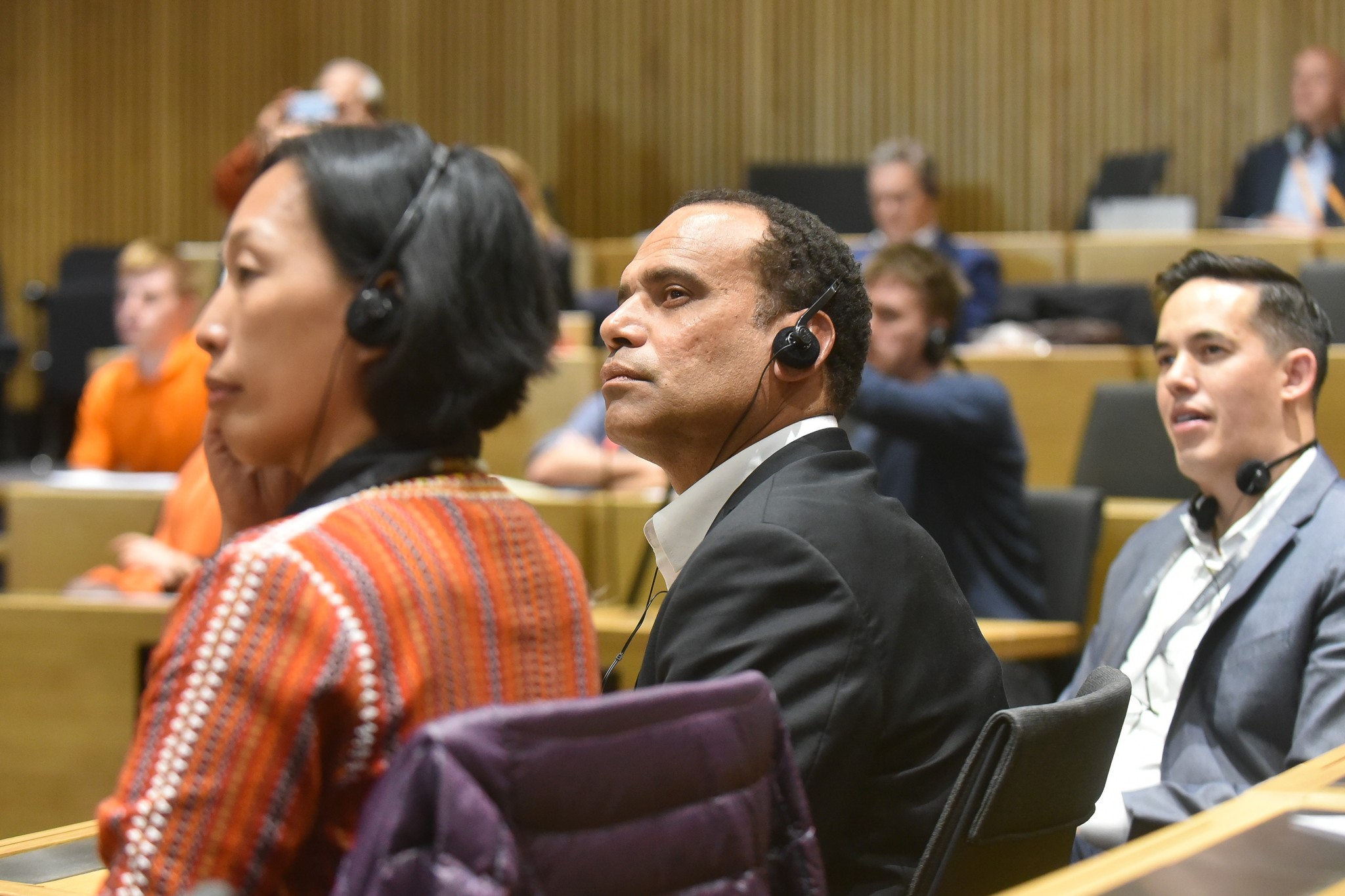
[847,144,1002,349]
[1060,252,1345,857]
[64,236,216,472]
[83,442,229,595]
[835,238,1056,708]
[477,146,574,310]
[95,124,602,896]
[526,391,669,492]
[211,58,382,218]
[603,188,1012,896]
[1220,48,1345,228]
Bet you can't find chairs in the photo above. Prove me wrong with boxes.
[1077,384,1203,497]
[330,670,831,896]
[907,664,1133,896]
[999,282,1148,345]
[1026,486,1102,621]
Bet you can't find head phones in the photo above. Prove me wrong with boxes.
[1191,442,1309,528]
[771,260,854,368]
[346,144,450,347]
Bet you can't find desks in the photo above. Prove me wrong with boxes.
[0,231,1345,896]
